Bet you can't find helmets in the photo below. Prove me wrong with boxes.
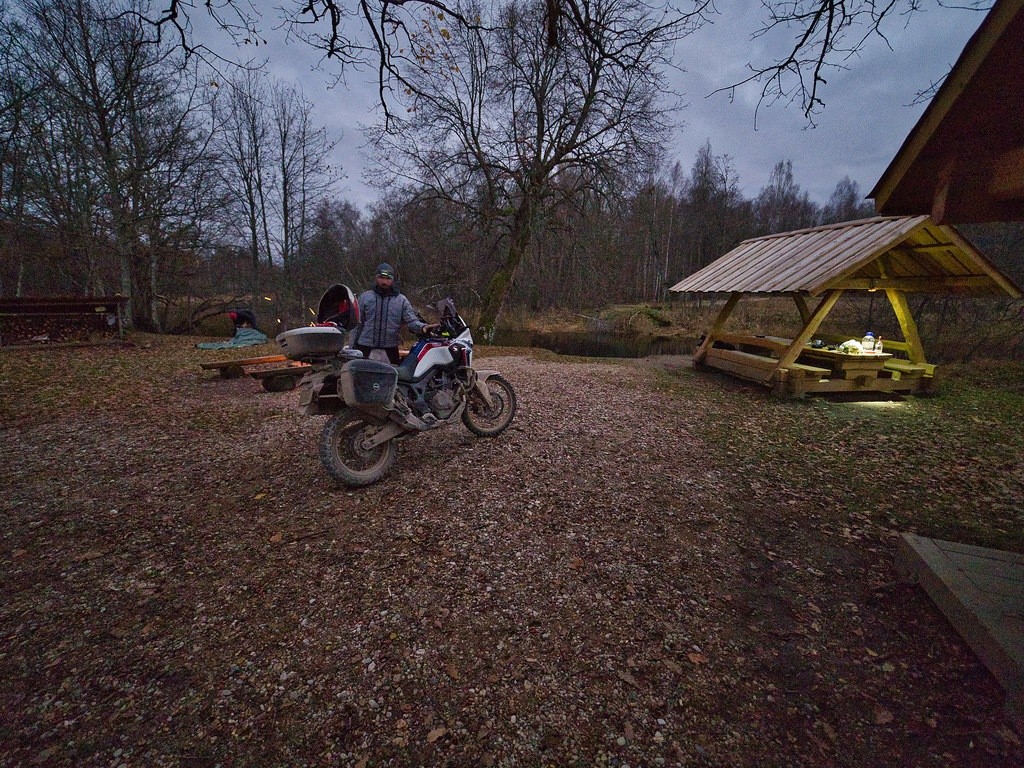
[373,262,395,280]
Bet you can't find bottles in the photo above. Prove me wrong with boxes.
[862,332,875,353]
[875,335,883,355]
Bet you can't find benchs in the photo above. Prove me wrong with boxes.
[787,333,927,375]
[198,353,288,370]
[247,365,314,378]
[697,345,831,375]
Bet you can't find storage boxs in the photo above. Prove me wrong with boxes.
[275,282,360,361]
[340,358,398,407]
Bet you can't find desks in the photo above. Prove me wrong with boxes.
[713,333,890,378]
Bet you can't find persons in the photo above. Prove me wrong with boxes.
[350,263,440,365]
[228,310,258,337]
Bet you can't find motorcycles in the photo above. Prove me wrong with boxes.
[276,283,517,488]
